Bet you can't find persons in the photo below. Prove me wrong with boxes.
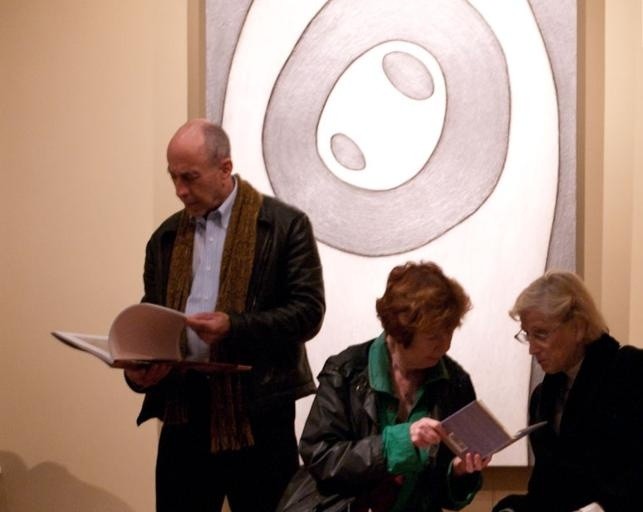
[121,116,328,511]
[275,258,493,512]
[489,272,642,511]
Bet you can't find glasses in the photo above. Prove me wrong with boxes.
[514,321,563,344]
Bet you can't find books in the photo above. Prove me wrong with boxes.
[48,301,191,369]
[431,398,546,463]
